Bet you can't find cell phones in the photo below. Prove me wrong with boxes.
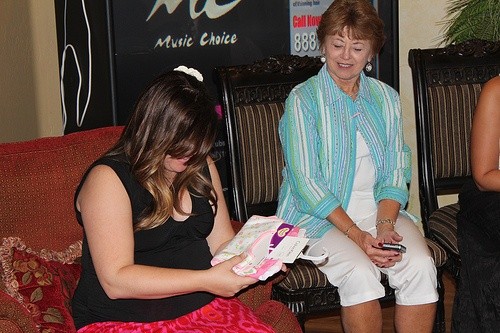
[381,243,406,254]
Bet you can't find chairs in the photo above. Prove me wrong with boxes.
[408,39,500,282]
[215,52,449,333]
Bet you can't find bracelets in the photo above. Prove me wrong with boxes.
[375,218,396,228]
[345,223,357,238]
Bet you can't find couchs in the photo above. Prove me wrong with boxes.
[0,125,300,333]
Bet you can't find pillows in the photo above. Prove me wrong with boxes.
[0,236,82,333]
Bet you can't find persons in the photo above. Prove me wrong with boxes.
[275,1,439,333]
[70,71,278,333]
[450,75,500,333]
